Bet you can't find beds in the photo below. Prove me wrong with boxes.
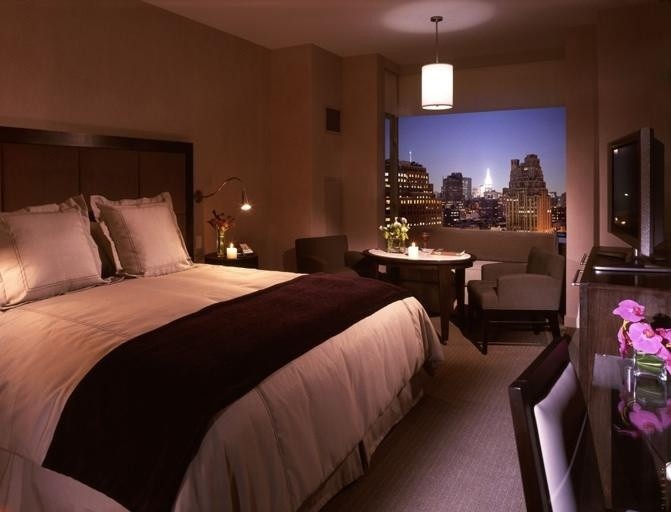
[0,125,452,509]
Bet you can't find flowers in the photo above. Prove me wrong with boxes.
[380,216,410,241]
[612,300,671,372]
[208,209,236,232]
[616,386,671,439]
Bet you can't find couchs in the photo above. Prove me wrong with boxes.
[396,226,558,318]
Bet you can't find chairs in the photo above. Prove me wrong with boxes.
[468,247,567,355]
[297,234,374,278]
[510,333,610,512]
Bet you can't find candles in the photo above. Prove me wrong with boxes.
[227,243,238,259]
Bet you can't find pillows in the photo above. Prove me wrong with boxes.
[0,192,112,313]
[90,190,198,278]
[87,219,113,279]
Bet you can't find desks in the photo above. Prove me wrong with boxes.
[364,248,475,343]
[549,353,671,511]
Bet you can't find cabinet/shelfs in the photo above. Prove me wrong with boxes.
[573,247,671,512]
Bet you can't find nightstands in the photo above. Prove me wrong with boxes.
[207,252,258,268]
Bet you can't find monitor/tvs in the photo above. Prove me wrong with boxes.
[592,127,671,272]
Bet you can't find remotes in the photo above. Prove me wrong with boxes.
[597,251,628,258]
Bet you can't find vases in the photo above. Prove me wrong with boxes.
[388,238,406,252]
[634,375,666,408]
[217,227,225,256]
[634,349,669,380]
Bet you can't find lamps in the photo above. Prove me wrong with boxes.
[196,176,251,211]
[421,16,455,111]
